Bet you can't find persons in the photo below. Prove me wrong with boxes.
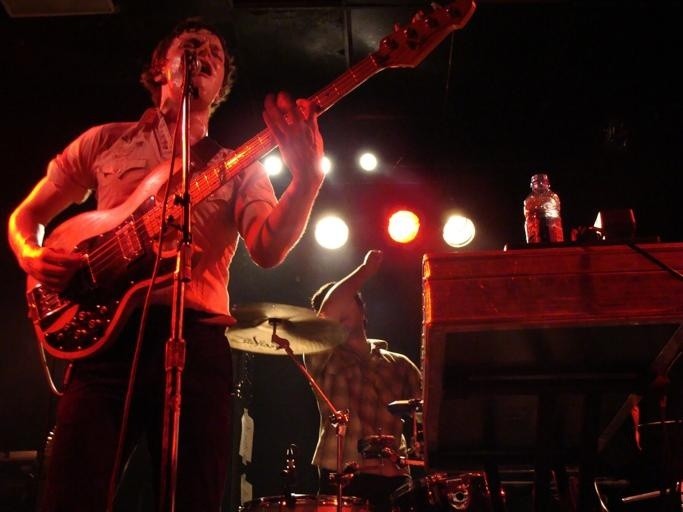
[521,170,567,247]
[5,15,328,509]
[299,249,424,510]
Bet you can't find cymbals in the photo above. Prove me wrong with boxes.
[225,302,350,356]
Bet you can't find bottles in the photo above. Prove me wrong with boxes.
[522,174,563,244]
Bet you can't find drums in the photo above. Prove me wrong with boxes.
[390,472,493,512]
[241,493,371,512]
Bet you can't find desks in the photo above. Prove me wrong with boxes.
[410,240,681,446]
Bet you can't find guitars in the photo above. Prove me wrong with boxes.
[25,1,477,361]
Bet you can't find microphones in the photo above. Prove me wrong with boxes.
[181,49,201,73]
